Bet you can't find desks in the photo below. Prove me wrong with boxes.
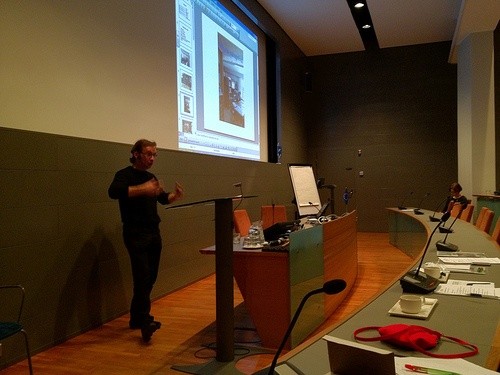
[249,204,499,375]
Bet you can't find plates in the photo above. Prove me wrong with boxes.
[388,298,438,320]
[417,268,450,282]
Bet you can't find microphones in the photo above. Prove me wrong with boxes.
[271,201,277,227]
[399,191,468,294]
[267,279,347,375]
[234,182,241,212]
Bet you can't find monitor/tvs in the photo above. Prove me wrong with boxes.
[287,163,321,216]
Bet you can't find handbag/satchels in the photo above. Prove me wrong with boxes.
[353,324,479,359]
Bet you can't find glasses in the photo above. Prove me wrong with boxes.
[140,151,156,157]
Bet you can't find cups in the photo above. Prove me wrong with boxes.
[400,295,426,314]
[424,266,440,279]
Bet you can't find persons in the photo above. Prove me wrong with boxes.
[108,138,185,343]
[441,182,468,212]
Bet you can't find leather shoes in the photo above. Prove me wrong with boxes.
[142,321,161,343]
[129,316,154,329]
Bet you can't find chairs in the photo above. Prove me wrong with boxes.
[449,201,463,219]
[479,210,495,234]
[459,204,474,224]
[475,206,489,229]
[233,209,253,238]
[0,284,34,375]
[491,217,500,242]
[272,204,288,226]
[260,205,273,232]
